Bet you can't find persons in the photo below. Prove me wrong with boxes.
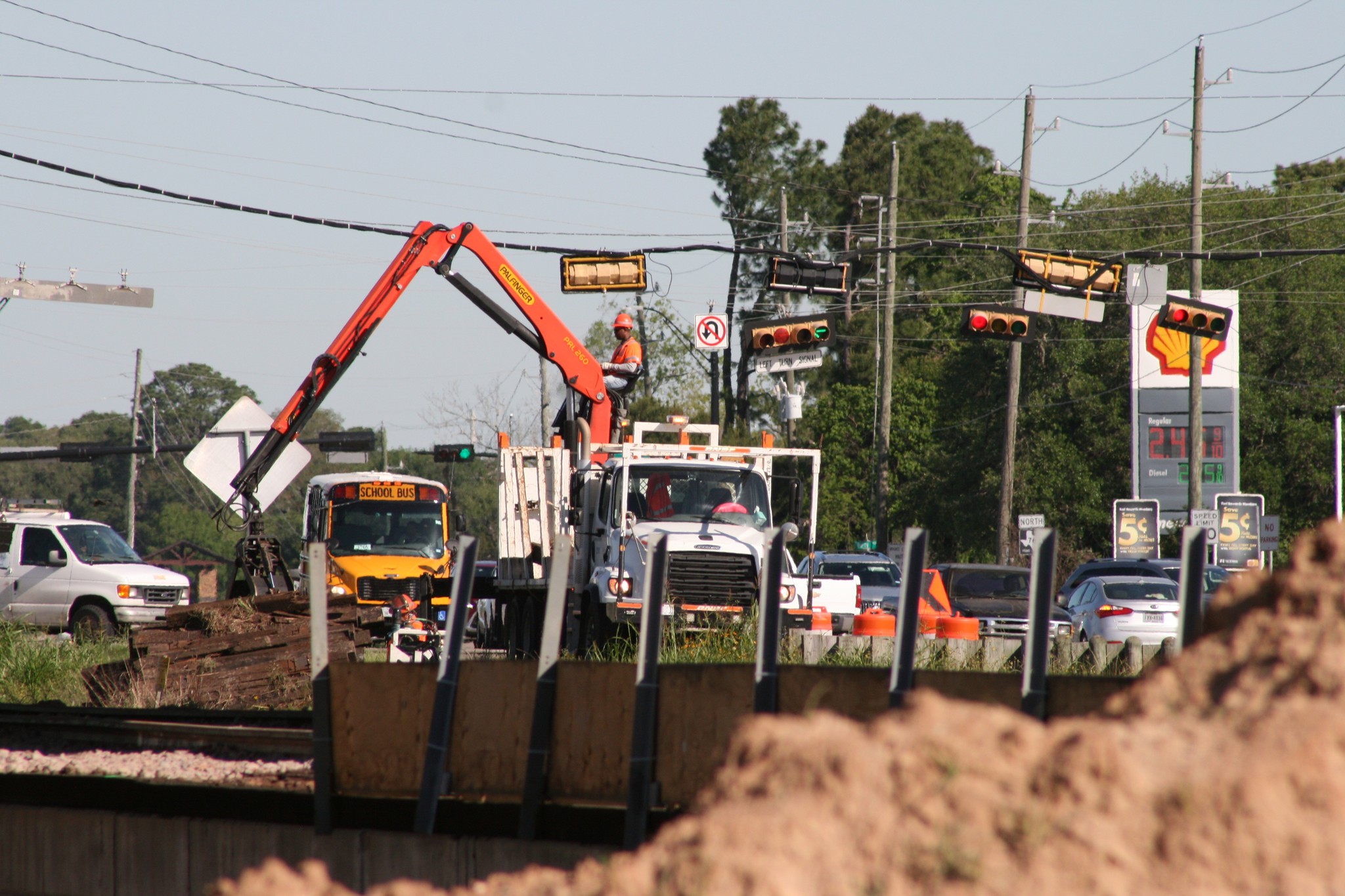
[387,594,427,665]
[579,314,642,421]
[1004,575,1021,595]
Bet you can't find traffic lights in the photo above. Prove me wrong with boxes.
[432,444,475,464]
[960,305,1030,340]
[743,315,835,352]
[1159,295,1232,344]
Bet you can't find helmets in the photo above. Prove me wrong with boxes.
[613,314,633,328]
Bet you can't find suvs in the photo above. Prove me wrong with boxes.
[799,550,904,616]
[0,508,192,645]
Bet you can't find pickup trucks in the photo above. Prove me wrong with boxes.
[924,562,1075,644]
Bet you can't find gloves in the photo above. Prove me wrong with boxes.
[600,362,608,369]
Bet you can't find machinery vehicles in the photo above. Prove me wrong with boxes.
[229,218,612,598]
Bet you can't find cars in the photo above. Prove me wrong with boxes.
[1056,559,1236,666]
[466,561,501,620]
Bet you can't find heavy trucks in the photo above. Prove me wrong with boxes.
[295,471,465,630]
[495,414,822,661]
